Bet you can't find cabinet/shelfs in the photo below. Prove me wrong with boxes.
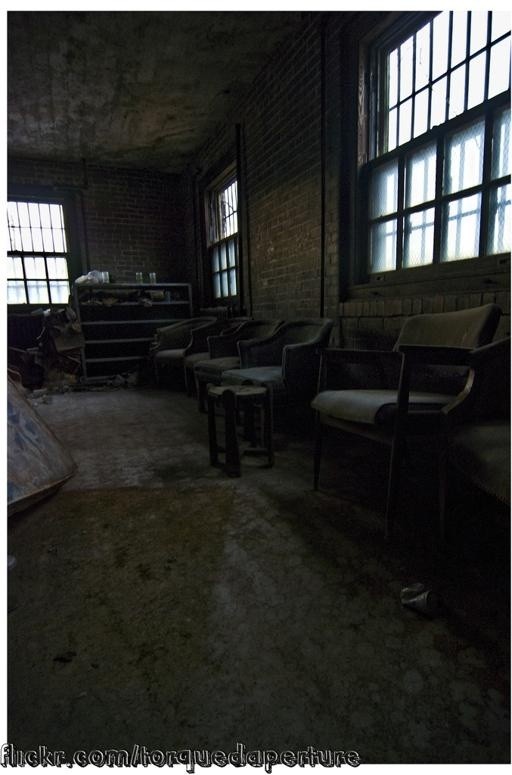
[78,282,194,385]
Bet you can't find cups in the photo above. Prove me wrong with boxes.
[164,291,171,302]
[150,272,156,284]
[136,272,143,284]
[101,271,110,284]
